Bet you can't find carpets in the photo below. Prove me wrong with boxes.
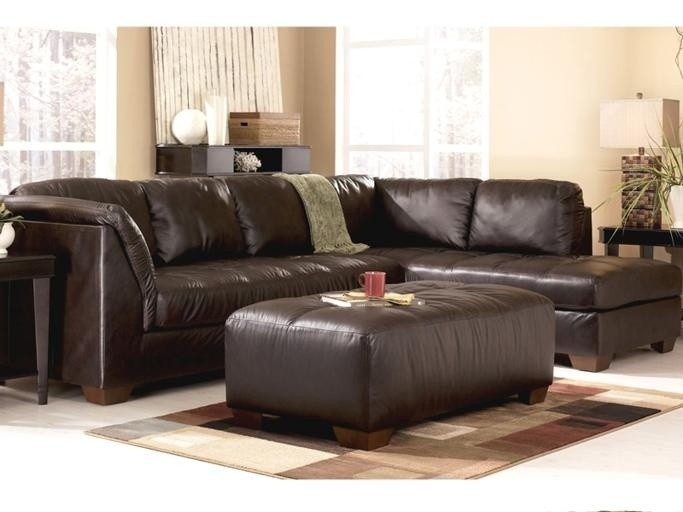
[85,377,683,482]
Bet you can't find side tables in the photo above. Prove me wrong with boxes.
[596,224,683,261]
[0,250,64,406]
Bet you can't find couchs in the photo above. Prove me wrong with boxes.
[0,172,682,406]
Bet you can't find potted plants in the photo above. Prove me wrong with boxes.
[0,199,26,260]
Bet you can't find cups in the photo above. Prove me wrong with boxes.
[358,271,386,299]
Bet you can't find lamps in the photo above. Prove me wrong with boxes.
[596,93,679,225]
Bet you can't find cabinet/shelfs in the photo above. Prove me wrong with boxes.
[153,141,312,175]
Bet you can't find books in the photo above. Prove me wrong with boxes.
[319,292,394,310]
[342,290,415,306]
